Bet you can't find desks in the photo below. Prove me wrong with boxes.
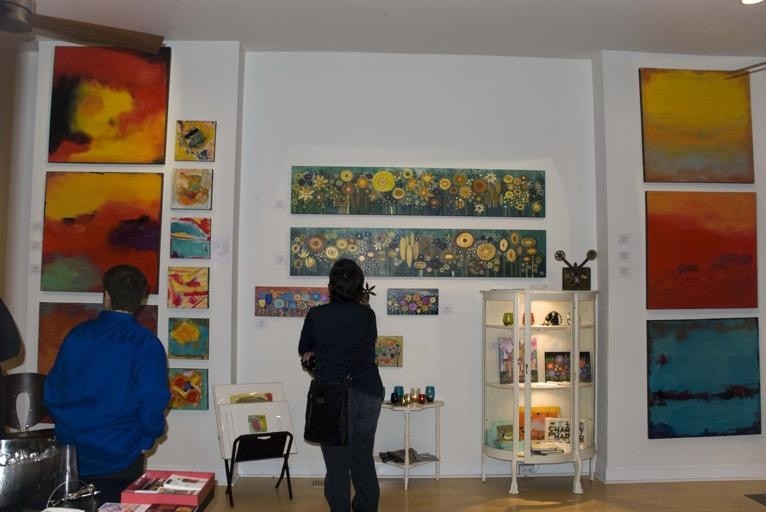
[374,400,446,491]
[1,472,216,511]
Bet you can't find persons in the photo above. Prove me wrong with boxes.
[42,263,171,482]
[297,258,386,512]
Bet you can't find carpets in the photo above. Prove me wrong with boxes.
[743,493,766,506]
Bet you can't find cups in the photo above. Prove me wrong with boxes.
[522,312,534,326]
[503,313,515,325]
[390,385,435,405]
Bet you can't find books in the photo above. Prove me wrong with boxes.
[484,405,587,456]
[97,472,209,512]
[545,351,592,382]
[498,336,539,384]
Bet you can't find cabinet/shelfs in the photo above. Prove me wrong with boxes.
[478,287,599,496]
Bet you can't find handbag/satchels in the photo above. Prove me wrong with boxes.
[304,379,350,449]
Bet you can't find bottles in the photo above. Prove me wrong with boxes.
[54,445,80,492]
